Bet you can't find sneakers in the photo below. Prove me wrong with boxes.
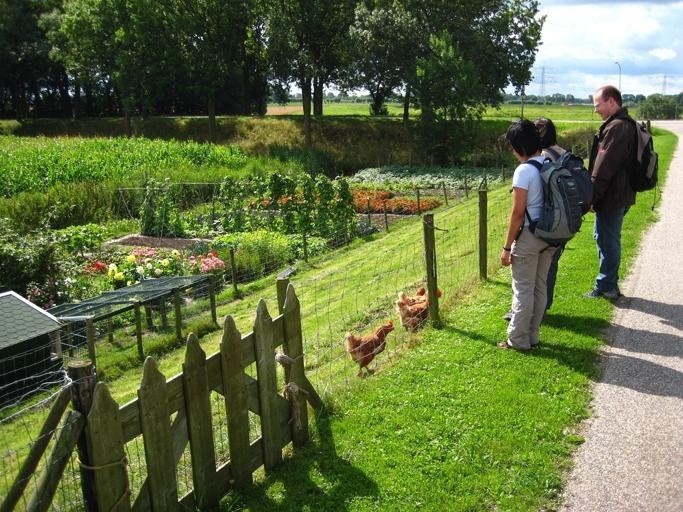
[501,309,514,322]
[582,284,621,301]
[496,339,541,353]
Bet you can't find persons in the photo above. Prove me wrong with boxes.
[494,118,560,353]
[583,85,637,302]
[503,118,567,322]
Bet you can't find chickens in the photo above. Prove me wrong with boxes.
[397,286,442,333]
[345,320,395,378]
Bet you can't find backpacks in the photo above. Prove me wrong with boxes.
[524,158,583,249]
[543,146,594,217]
[613,114,659,193]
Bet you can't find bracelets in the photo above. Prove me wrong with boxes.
[502,246,511,251]
